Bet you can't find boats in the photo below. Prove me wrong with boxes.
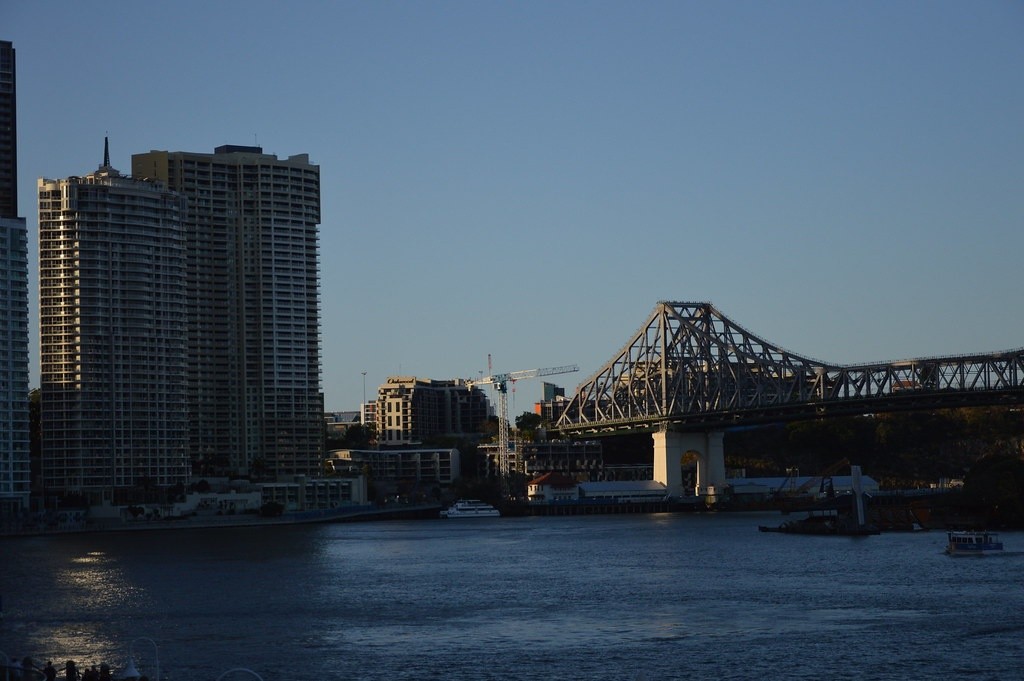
[946,529,1006,556]
[439,499,501,519]
[758,506,882,540]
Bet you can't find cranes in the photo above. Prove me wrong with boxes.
[468,363,581,480]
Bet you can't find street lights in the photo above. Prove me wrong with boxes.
[361,371,369,436]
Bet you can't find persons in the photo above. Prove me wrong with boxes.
[44,660,148,681]
